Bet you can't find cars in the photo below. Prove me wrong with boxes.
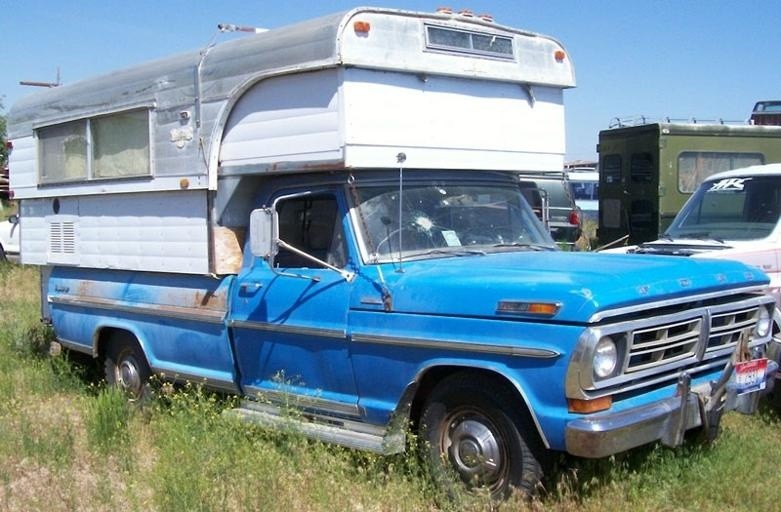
[1,214,19,265]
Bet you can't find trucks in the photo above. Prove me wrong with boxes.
[7,5,780,507]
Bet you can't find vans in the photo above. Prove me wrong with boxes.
[596,103,780,250]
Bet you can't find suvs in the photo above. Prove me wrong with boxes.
[518,172,585,249]
[595,162,781,378]
